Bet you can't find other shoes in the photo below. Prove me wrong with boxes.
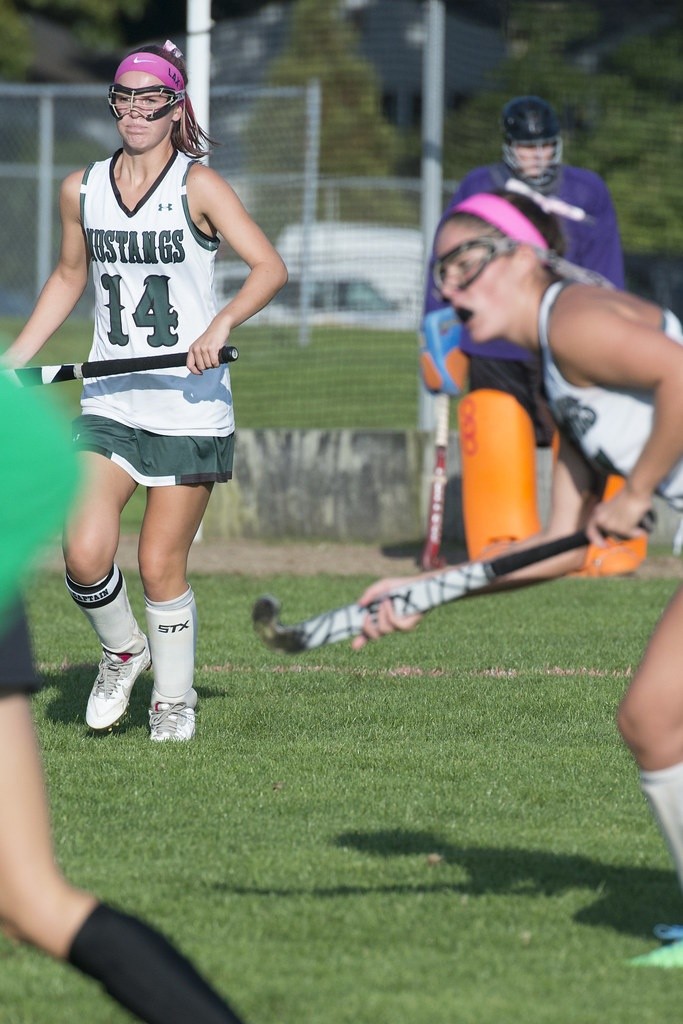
[624,923,683,968]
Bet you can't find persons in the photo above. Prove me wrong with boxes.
[0,357,247,1024]
[419,95,626,449]
[351,188,683,969]
[0,40,288,742]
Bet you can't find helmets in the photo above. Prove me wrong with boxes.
[502,96,563,195]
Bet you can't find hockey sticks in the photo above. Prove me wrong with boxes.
[422,393,450,571]
[1,345,240,390]
[252,511,656,655]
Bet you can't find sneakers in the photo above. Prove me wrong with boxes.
[86,632,152,729]
[148,701,198,744]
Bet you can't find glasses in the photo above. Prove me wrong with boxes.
[432,236,520,303]
[108,84,185,121]
